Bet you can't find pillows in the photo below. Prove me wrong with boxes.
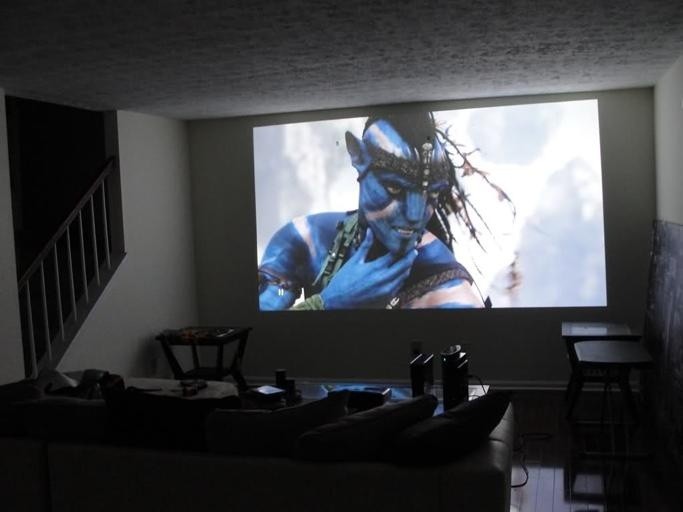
[34,367,77,395]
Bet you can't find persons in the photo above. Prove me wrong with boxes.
[258,107,512,309]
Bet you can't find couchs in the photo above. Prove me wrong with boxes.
[0,376,515,511]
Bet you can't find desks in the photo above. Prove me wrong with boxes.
[154,325,253,391]
[560,321,655,499]
